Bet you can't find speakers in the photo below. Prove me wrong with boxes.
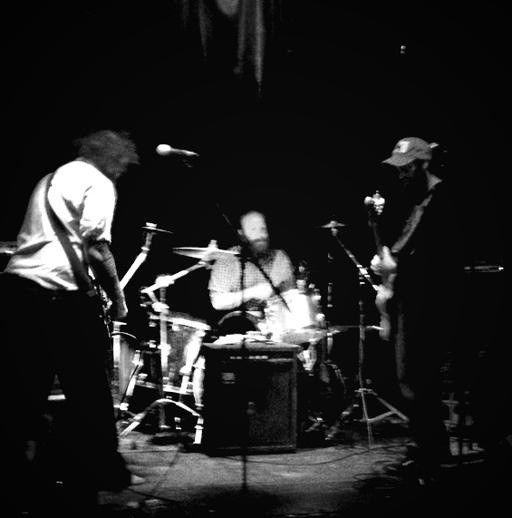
[205,344,299,458]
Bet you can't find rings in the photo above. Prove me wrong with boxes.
[378,267,381,273]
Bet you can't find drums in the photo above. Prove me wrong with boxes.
[132,309,211,396]
[263,288,329,344]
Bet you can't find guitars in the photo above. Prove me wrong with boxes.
[364,192,398,342]
[84,266,114,347]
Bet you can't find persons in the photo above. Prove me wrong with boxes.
[179,208,330,450]
[2,126,145,514]
[371,136,471,490]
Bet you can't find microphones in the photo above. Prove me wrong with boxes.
[155,143,198,157]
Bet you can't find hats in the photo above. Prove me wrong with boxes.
[380,136,433,167]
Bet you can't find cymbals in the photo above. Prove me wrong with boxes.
[172,246,238,261]
[311,222,345,230]
[142,226,174,234]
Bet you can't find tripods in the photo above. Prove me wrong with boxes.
[318,238,410,453]
[114,260,205,442]
